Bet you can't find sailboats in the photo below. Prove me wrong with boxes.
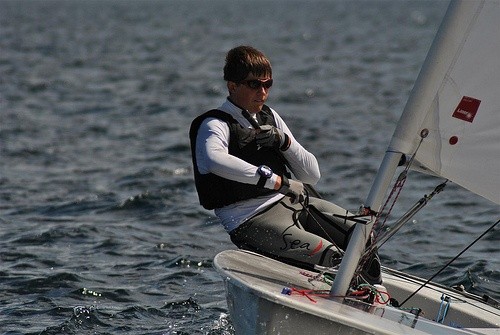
[211,1,500,335]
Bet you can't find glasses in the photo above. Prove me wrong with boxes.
[234,79,273,90]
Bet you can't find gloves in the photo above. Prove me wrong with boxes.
[278,175,309,205]
[255,125,285,149]
